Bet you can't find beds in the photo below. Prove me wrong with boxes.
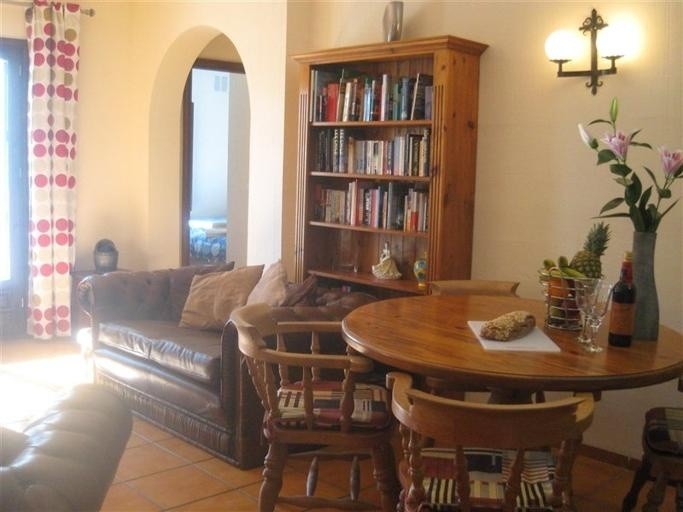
[188,215,227,262]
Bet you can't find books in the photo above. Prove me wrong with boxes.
[314,179,428,232]
[309,68,434,123]
[314,128,431,177]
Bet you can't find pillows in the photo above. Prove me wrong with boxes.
[176,264,263,332]
[247,260,287,309]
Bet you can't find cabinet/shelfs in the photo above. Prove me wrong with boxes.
[291,34,489,306]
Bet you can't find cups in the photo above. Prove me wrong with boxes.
[574,276,604,345]
[582,279,614,353]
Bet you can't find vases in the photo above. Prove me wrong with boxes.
[629,231,660,340]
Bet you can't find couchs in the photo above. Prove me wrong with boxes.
[75,261,424,470]
[2,382,133,512]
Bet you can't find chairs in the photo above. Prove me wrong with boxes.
[620,400,683,510]
[229,299,397,510]
[382,370,595,509]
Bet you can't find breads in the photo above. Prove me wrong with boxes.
[480,310,536,341]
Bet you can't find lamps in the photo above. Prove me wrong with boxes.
[545,8,639,96]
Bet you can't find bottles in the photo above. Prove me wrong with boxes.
[609,252,638,347]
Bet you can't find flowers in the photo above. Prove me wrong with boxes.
[577,103,683,229]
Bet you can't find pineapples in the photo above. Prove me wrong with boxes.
[567,220,613,298]
